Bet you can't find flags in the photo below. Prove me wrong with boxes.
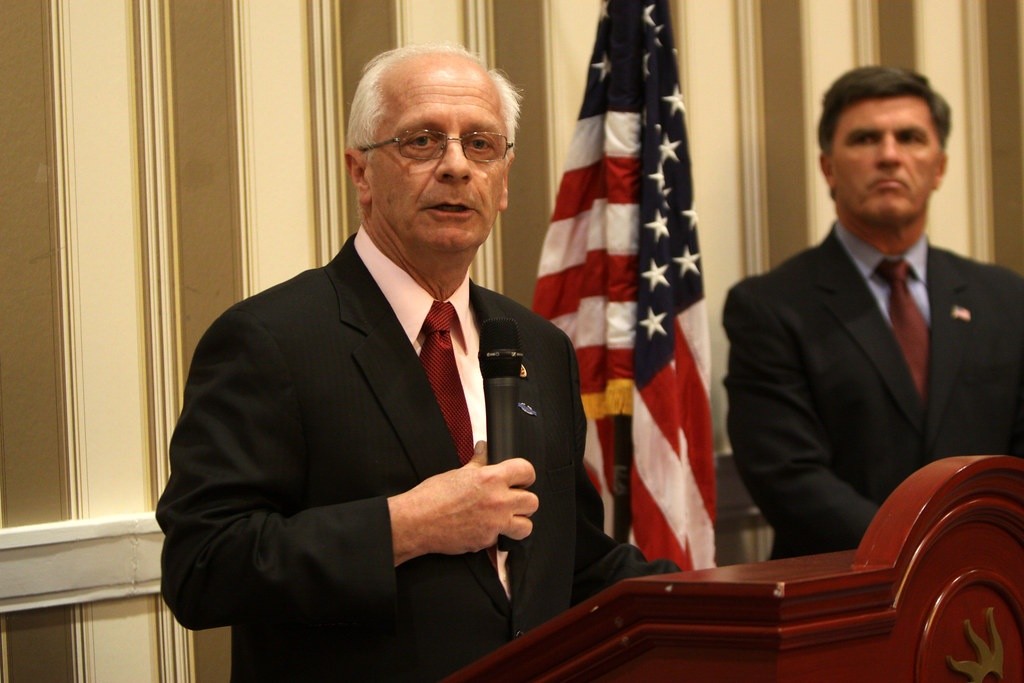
[529,1,716,572]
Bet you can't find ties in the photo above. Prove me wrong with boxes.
[873,257,933,410]
[416,301,498,579]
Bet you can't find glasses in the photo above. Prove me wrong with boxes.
[357,128,514,163]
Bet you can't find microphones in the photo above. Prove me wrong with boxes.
[478,317,524,551]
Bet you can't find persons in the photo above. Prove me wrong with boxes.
[156,41,681,683]
[716,63,1024,556]
